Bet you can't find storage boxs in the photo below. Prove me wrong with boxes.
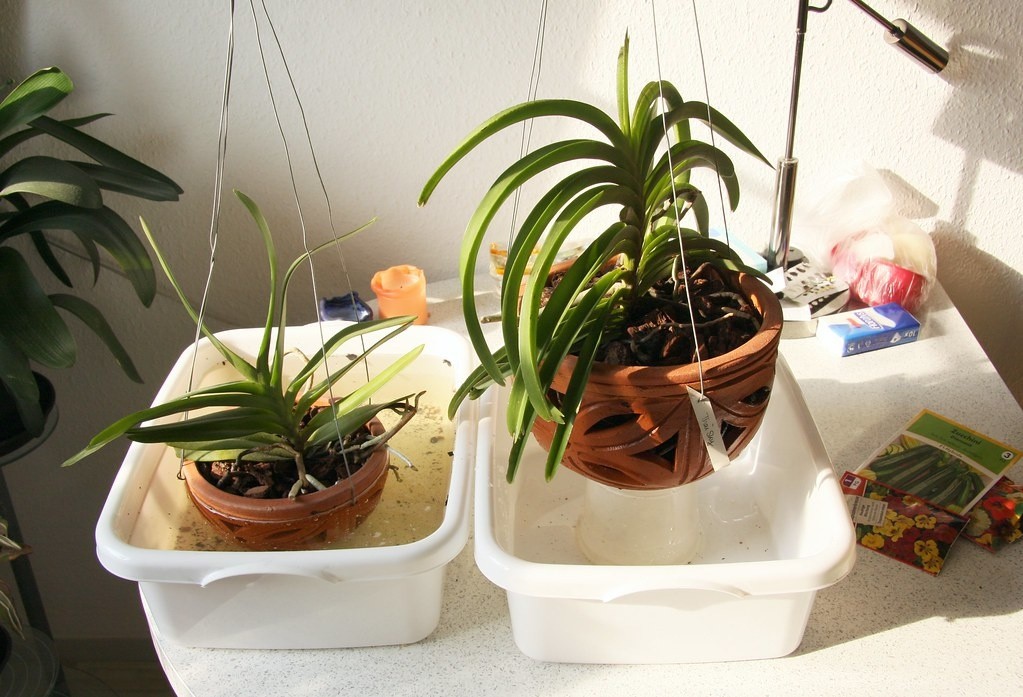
[95,320,480,650]
[471,335,860,661]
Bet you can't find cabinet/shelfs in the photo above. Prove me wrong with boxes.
[0,368,117,697]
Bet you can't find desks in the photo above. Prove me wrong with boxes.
[156,267,1023,697]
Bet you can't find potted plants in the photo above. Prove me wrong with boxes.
[60,189,426,546]
[419,26,785,492]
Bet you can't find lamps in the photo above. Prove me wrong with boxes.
[753,0,950,317]
